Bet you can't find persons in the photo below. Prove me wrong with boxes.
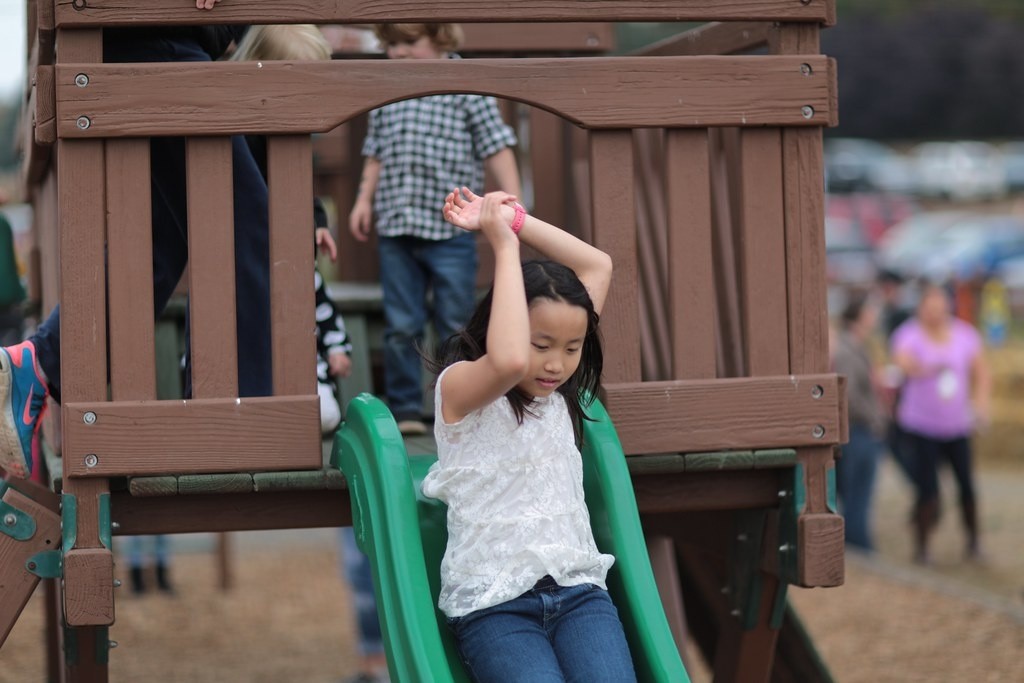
[305,231,352,438]
[420,184,634,683]
[186,22,339,427]
[127,535,176,597]
[348,20,520,439]
[831,256,1013,574]
[340,527,394,683]
[0,1,303,483]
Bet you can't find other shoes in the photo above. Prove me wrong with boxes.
[398,418,426,434]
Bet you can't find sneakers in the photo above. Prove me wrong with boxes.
[1,340,51,479]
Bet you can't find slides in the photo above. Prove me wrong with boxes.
[331,382,694,683]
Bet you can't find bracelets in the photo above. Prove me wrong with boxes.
[507,202,526,235]
